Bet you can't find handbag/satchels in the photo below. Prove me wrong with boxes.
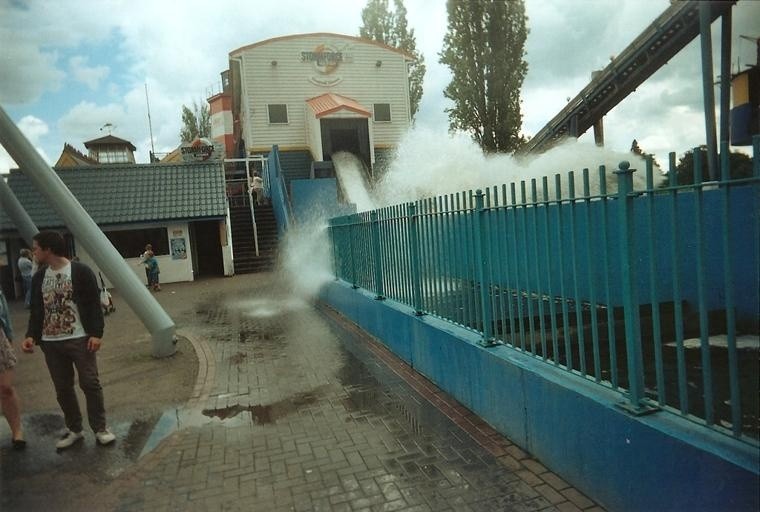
[99,285,111,308]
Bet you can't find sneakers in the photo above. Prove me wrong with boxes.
[54,430,85,449]
[94,429,117,446]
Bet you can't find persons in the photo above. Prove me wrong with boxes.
[0,289,27,448]
[137,252,161,292]
[22,249,33,309]
[139,244,153,286]
[251,175,262,206]
[253,171,264,207]
[18,248,26,270]
[20,230,116,448]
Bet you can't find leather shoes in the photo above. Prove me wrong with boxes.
[11,436,27,447]
